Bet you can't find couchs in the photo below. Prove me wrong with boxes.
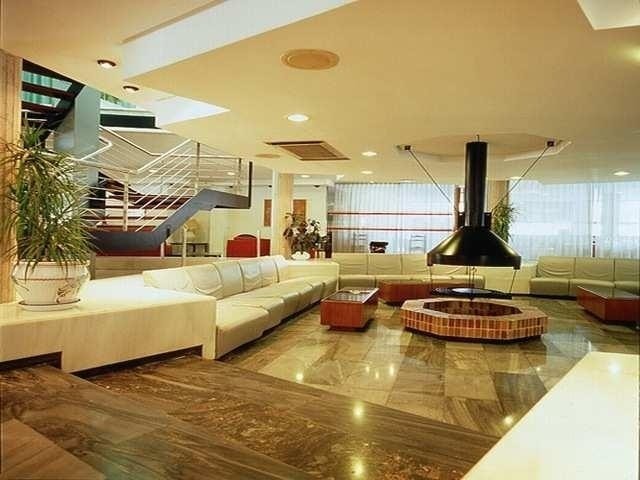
[529,256,639,301]
[332,253,485,303]
[140,254,337,358]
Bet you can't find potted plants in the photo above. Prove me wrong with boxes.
[284,211,321,259]
[0,117,93,312]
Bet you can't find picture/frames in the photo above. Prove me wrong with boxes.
[264,199,307,228]
